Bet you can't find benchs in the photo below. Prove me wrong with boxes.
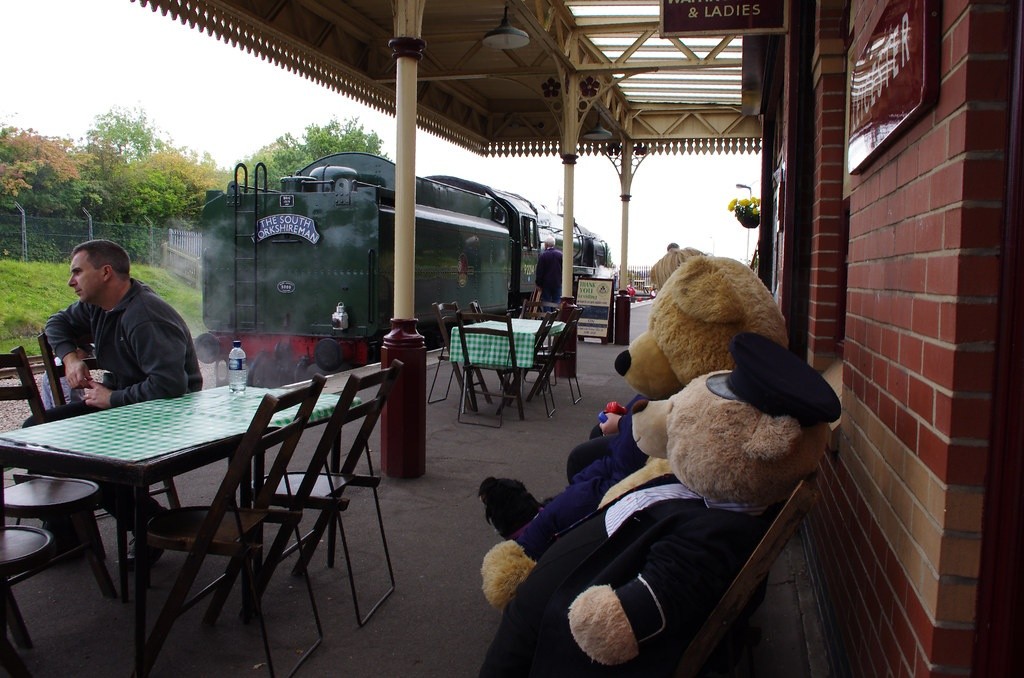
[671,477,821,678]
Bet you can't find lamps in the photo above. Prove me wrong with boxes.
[482,6,530,49]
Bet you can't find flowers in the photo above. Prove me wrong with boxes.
[728,197,761,217]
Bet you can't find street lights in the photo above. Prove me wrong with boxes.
[736,183,751,262]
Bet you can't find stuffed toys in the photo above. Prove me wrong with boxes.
[477,255,842,678]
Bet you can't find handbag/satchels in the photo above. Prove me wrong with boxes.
[478,475,544,540]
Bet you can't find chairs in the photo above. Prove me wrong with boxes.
[0,346,117,648]
[0,524,57,677]
[38,333,181,560]
[427,299,585,429]
[243,357,405,627]
[130,373,326,678]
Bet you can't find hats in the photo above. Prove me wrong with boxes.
[707,328,841,428]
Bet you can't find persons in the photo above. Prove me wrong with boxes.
[534,235,563,313]
[42,342,95,411]
[21,239,203,573]
[567,244,708,485]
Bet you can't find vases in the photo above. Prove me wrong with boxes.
[737,214,759,228]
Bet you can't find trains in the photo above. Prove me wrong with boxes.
[193,152,617,389]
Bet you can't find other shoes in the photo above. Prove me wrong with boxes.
[127,504,170,571]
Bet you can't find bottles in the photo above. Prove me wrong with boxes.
[228,340,247,392]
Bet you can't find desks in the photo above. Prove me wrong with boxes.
[0,385,362,677]
[450,319,567,413]
